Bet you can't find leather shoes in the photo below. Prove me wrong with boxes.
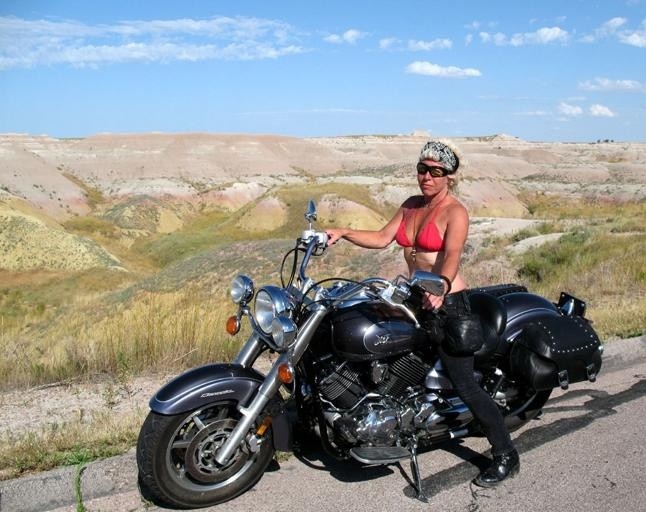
[476,449,520,488]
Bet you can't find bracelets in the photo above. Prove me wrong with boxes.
[437,274,452,298]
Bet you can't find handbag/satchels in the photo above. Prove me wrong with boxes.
[443,314,484,353]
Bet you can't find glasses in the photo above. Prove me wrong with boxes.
[417,163,448,177]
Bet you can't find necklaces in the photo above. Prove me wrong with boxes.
[409,195,446,264]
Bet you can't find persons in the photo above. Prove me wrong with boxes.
[324,142,520,488]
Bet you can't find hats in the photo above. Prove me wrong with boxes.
[420,141,459,173]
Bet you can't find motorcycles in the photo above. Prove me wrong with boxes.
[136,201,586,508]
